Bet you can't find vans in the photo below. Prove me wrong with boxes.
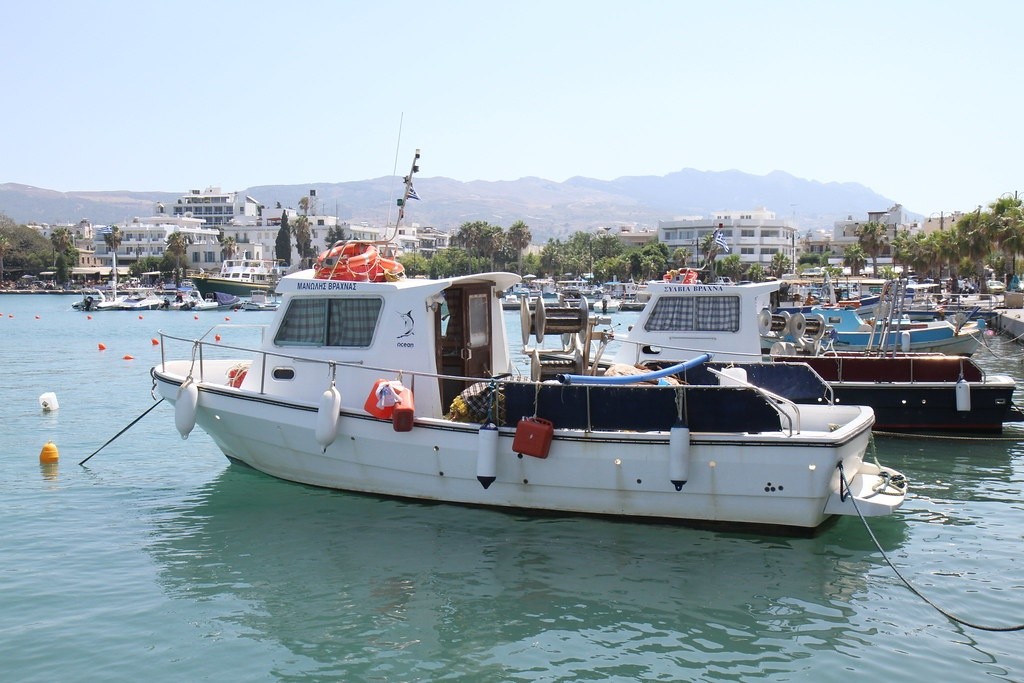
[32,281,46,287]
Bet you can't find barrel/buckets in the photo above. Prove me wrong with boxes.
[392,386,414,432]
[364,378,398,419]
[512,416,555,459]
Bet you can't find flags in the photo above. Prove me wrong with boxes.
[716,231,730,253]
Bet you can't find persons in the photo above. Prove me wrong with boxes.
[0,279,104,290]
[963,279,970,296]
[950,274,959,303]
[804,292,814,306]
[176,290,183,303]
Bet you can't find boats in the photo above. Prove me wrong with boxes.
[149,110,911,538]
[501,271,1000,357]
[71,281,282,312]
[186,249,284,301]
[528,222,1024,436]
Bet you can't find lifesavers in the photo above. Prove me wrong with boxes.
[319,244,377,268]
[663,273,698,280]
[370,256,405,273]
[665,279,697,284]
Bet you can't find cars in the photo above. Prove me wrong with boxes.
[986,281,1007,295]
